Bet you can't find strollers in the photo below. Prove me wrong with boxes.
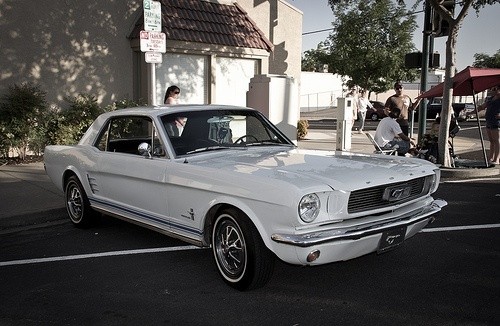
[413,117,459,167]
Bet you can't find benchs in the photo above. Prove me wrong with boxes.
[108,136,185,153]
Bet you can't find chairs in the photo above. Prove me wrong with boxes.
[366,133,398,155]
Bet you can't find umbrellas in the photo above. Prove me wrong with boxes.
[414,66,500,167]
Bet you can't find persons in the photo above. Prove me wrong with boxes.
[346,90,357,136]
[163,86,185,136]
[374,108,417,156]
[384,82,424,138]
[467,86,500,164]
[357,90,377,134]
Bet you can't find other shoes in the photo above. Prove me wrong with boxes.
[358,129,363,133]
[351,132,354,137]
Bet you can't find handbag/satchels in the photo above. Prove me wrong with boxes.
[449,124,459,138]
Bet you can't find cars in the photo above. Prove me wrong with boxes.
[366,101,388,121]
[43,104,449,290]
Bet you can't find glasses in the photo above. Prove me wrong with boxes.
[396,86,403,91]
[174,91,179,94]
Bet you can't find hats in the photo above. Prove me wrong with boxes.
[394,82,403,86]
